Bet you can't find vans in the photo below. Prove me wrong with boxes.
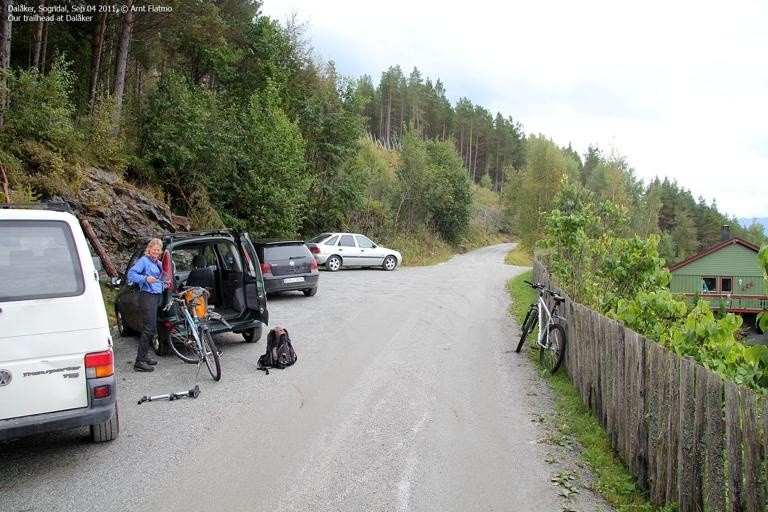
[0,201,119,444]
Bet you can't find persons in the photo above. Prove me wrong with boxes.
[126,237,170,372]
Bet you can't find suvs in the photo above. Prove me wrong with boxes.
[115,226,269,357]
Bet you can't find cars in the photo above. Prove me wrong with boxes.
[306,232,402,272]
[213,238,319,297]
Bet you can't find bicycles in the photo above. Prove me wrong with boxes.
[163,286,221,383]
[516,280,567,377]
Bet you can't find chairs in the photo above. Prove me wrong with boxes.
[186,255,217,303]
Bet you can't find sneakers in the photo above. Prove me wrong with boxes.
[147,359,157,365]
[134,360,154,371]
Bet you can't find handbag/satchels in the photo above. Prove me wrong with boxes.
[185,288,206,318]
[259,327,297,368]
